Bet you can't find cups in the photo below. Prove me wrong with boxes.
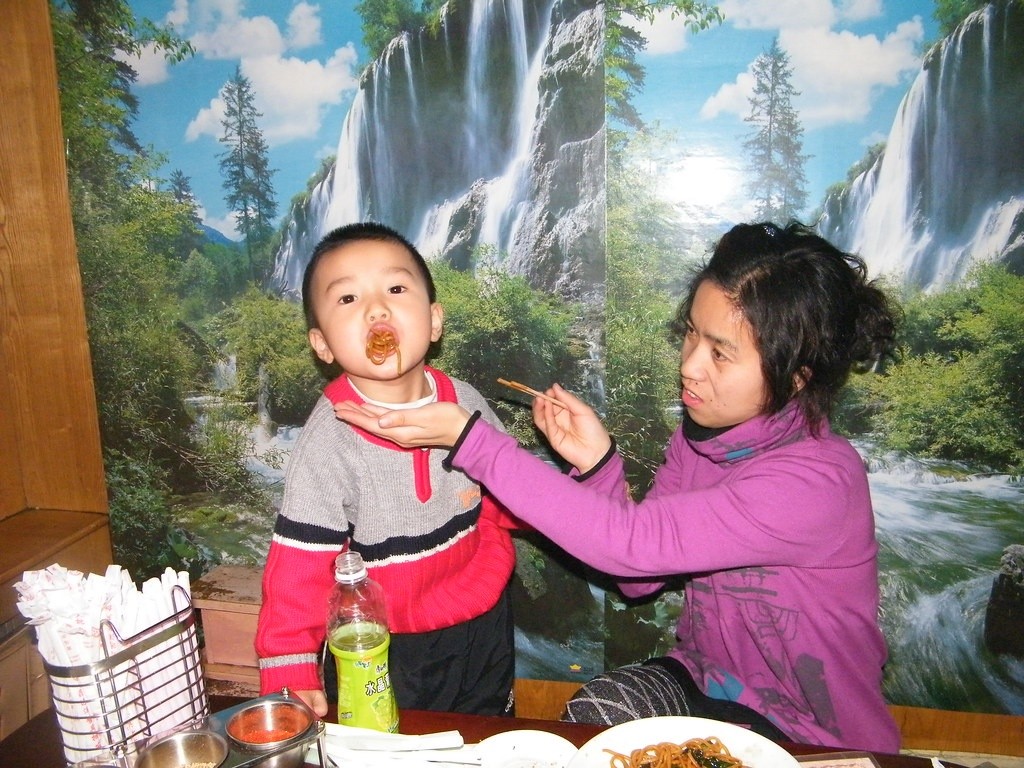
[225,700,313,768]
[134,730,228,768]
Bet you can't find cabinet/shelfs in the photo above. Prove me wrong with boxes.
[186,565,265,685]
[0,0,116,743]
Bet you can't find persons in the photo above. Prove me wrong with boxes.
[331,216,903,759]
[251,221,536,719]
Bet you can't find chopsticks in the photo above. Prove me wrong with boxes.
[496,378,567,410]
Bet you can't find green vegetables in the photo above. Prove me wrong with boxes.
[642,748,733,768]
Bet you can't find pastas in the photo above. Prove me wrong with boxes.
[366,327,401,376]
[601,736,747,768]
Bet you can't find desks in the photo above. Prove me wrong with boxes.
[0,691,974,768]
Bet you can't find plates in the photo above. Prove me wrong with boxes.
[476,729,578,767]
[575,716,801,768]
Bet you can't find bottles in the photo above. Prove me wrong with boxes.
[327,551,400,735]
